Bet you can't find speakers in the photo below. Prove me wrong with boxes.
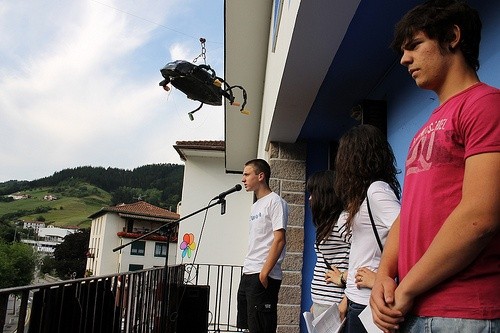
[172,285,210,333]
[351,99,387,142]
[319,140,339,172]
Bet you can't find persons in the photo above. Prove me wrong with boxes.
[369,1,500,333]
[337,125,402,333]
[306,170,352,333]
[237,159,288,332]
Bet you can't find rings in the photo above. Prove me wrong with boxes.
[360,275,362,282]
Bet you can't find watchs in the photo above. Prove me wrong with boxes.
[340,272,347,286]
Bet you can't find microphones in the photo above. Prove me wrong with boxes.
[219,184,242,199]
